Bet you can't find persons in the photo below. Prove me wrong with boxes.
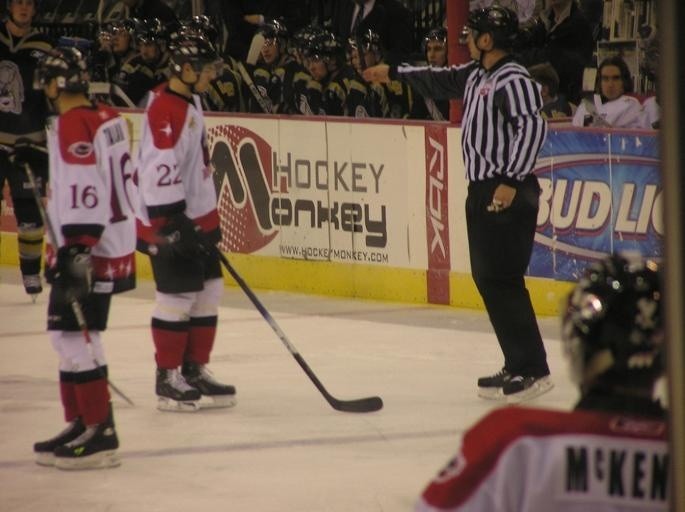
[360,6,558,403]
[138,34,238,410]
[30,44,139,471]
[97,0,660,133]
[0,1,56,301]
[413,248,673,512]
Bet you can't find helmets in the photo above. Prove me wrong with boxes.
[461,8,522,50]
[563,259,666,381]
[423,28,448,58]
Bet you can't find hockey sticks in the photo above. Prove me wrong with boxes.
[22,161,137,409]
[209,244,382,413]
[246,33,270,113]
[87,82,135,109]
[582,68,611,128]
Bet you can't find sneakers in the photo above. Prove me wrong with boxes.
[33,415,84,452]
[22,273,42,294]
[476,368,511,388]
[502,372,544,395]
[55,406,121,458]
[157,368,200,400]
[179,364,237,395]
